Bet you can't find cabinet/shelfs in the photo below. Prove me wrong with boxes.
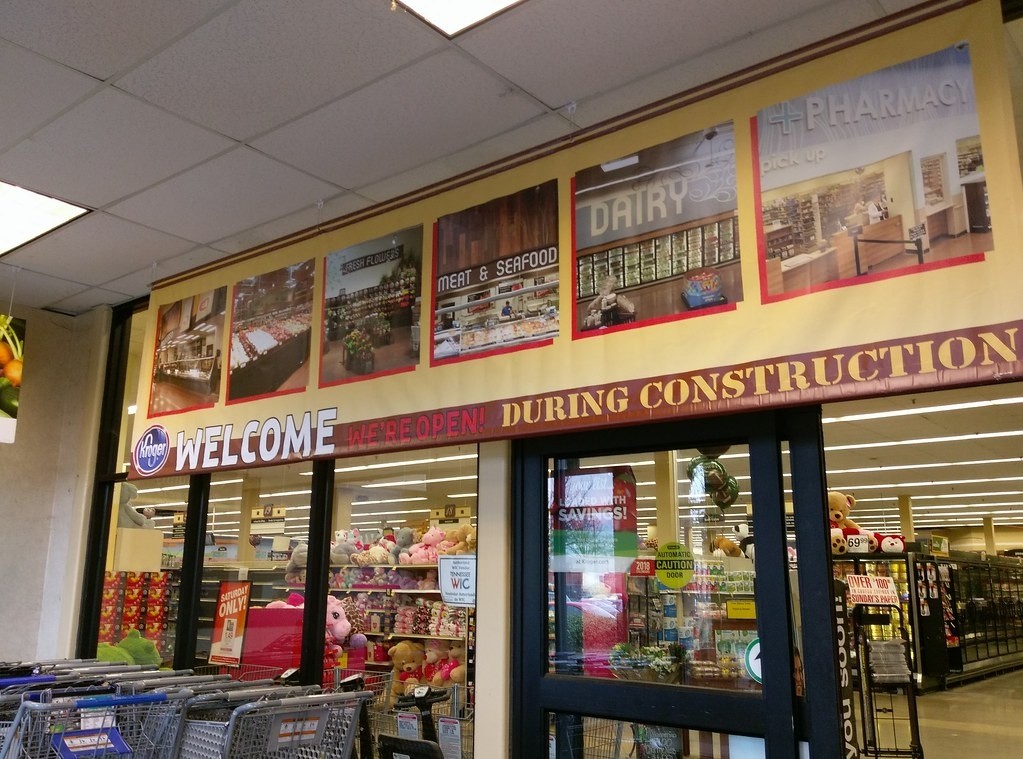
[627,558,756,679]
[323,277,420,373]
[765,149,981,263]
[164,566,475,719]
[832,551,1023,695]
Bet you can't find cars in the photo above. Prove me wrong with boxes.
[549,599,623,620]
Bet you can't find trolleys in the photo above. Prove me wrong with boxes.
[0,659,374,759]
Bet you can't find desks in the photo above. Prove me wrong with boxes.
[682,291,728,311]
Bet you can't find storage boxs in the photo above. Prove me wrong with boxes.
[98,571,171,653]
[685,560,756,593]
[716,630,758,660]
[657,581,695,647]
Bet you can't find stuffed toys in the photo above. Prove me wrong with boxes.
[117,482,146,528]
[880,535,906,553]
[827,492,879,555]
[266,592,351,658]
[284,524,476,719]
[713,523,755,559]
